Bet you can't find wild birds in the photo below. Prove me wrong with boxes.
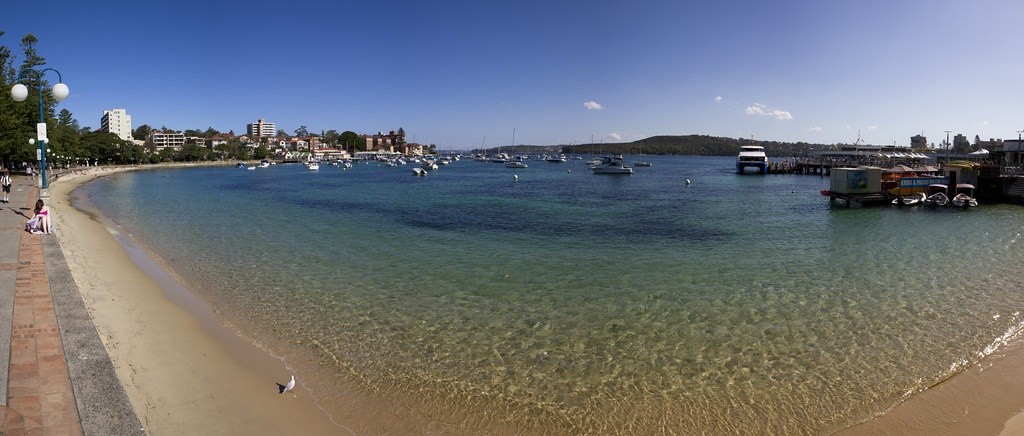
[282,375,295,394]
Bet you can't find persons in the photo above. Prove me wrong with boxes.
[26,199,52,234]
[0,158,100,182]
[1,168,12,204]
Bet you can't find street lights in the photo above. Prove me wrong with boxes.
[10,68,71,190]
[28,137,49,175]
[56,155,64,169]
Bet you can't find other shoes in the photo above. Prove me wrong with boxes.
[3,200,6,204]
[6,201,9,203]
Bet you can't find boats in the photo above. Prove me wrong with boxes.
[234,127,655,176]
[953,192,979,210]
[923,192,950,210]
[734,144,769,175]
[890,190,927,207]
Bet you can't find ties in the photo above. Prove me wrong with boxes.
[5,178,7,185]
